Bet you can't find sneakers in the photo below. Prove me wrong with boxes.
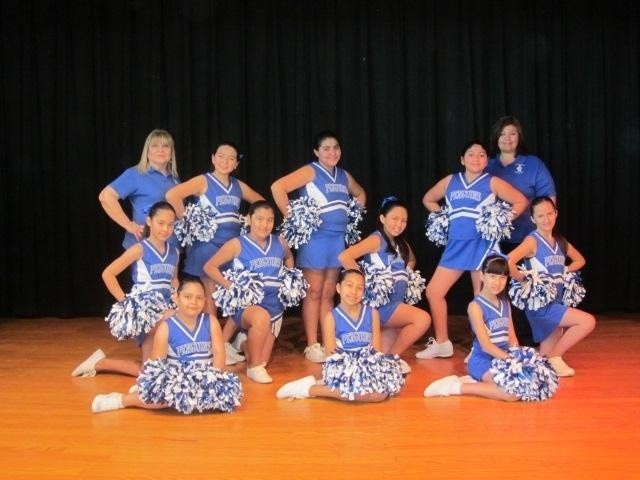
[71,349,105,379]
[547,356,575,377]
[415,337,454,359]
[92,391,125,414]
[424,374,478,397]
[225,331,325,399]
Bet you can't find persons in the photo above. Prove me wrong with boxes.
[484,115,558,351]
[337,196,432,376]
[98,127,183,293]
[71,201,183,377]
[277,269,391,402]
[423,253,529,402]
[204,200,297,386]
[164,141,269,368]
[270,131,369,363]
[413,139,530,361]
[508,195,598,377]
[92,274,226,412]
